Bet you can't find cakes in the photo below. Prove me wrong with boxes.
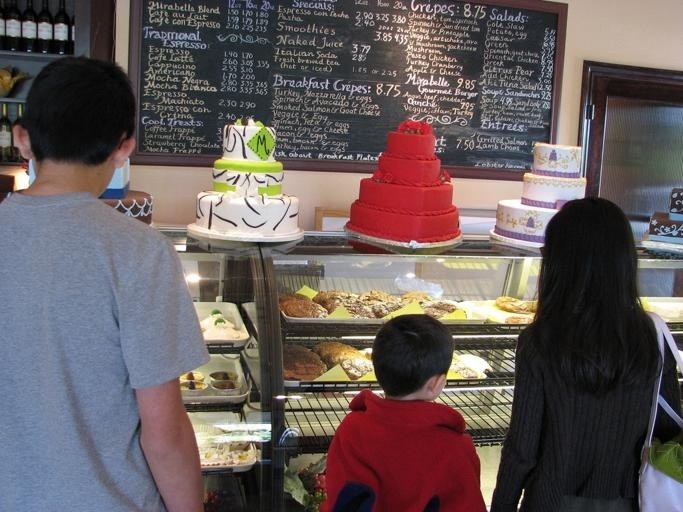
[494,142,589,244]
[194,120,303,239]
[98,157,153,230]
[344,123,461,243]
[639,187,682,255]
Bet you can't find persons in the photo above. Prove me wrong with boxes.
[320,314,487,512]
[490,197,683,511]
[0,56,211,512]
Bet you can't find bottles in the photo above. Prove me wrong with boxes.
[71,10,76,56]
[6,0,21,52]
[21,0,36,52]
[0,1,9,49]
[53,0,69,55]
[37,1,53,55]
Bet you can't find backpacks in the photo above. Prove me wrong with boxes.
[639,312,683,512]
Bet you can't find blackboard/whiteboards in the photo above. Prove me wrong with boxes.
[128,0,568,182]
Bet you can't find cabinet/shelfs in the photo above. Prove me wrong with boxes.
[0,0,116,166]
[258,237,683,507]
[149,226,272,510]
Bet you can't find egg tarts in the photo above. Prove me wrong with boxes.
[180,371,205,382]
[180,380,209,395]
[209,371,239,383]
[211,380,242,396]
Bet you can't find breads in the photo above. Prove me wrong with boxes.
[495,295,530,314]
[312,288,404,318]
[281,294,327,321]
[404,290,428,305]
[419,301,456,321]
[315,338,373,380]
[283,342,328,381]
[504,313,536,325]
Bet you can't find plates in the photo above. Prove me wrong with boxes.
[280,292,488,324]
[283,337,487,389]
[189,302,250,346]
[484,298,538,331]
[188,413,258,474]
[178,355,252,406]
